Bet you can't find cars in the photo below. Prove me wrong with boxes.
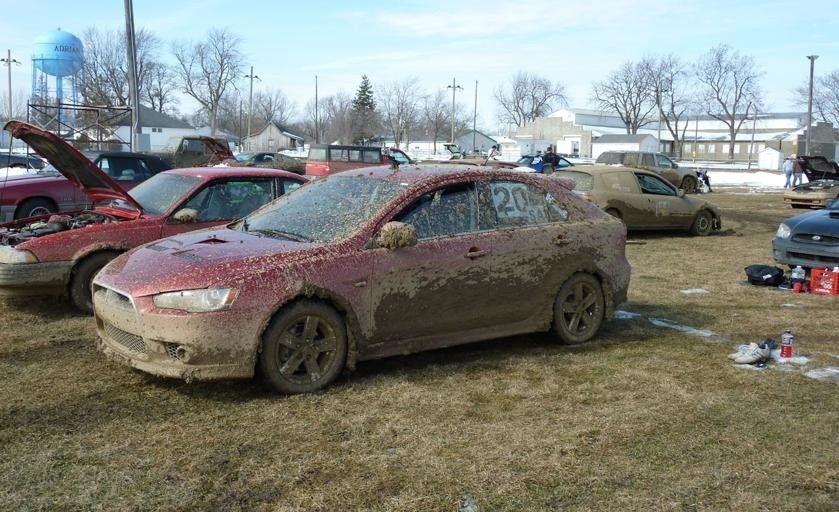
[771,192,839,272]
[781,153,839,210]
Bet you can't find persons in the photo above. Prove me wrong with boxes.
[488,145,501,160]
[544,147,560,166]
[783,156,803,188]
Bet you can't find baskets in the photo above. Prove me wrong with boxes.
[810,267,839,295]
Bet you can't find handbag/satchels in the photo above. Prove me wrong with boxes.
[744,265,784,287]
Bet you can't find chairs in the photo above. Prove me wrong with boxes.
[118,169,135,180]
[200,191,225,220]
[239,193,266,218]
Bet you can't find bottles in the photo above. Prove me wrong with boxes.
[830,267,839,272]
[792,265,805,286]
[780,330,793,357]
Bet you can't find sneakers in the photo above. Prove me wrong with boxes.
[727,341,769,364]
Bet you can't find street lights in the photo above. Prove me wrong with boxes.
[442,76,467,156]
[0,48,25,154]
[242,66,262,152]
[801,52,818,188]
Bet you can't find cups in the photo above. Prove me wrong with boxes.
[793,283,802,293]
